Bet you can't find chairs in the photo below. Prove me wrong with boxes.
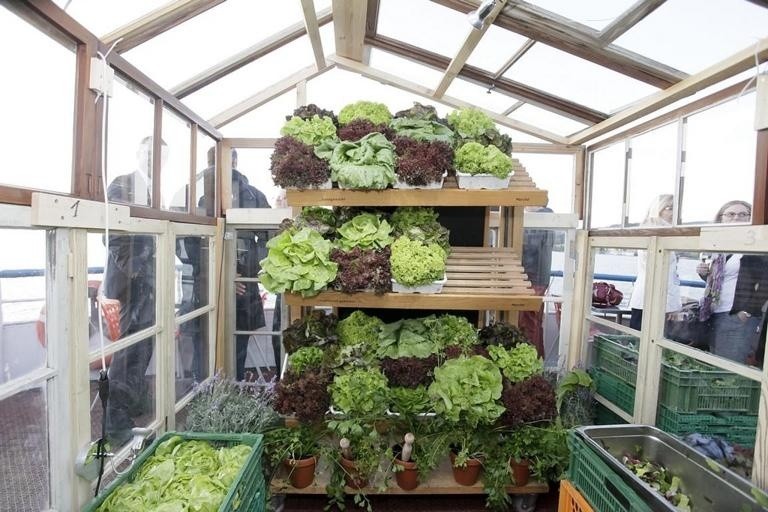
[244,290,273,384]
[89,299,129,415]
[542,291,564,370]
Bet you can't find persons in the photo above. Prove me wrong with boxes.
[103,137,178,442]
[697,200,768,363]
[629,193,683,337]
[173,146,278,381]
[523,205,554,361]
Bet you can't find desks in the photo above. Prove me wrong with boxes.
[591,297,632,327]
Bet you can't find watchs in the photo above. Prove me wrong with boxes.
[744,311,751,318]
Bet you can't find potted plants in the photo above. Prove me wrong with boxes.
[260,101,577,512]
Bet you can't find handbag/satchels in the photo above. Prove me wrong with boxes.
[591,281,623,308]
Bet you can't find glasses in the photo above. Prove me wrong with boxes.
[720,212,751,219]
